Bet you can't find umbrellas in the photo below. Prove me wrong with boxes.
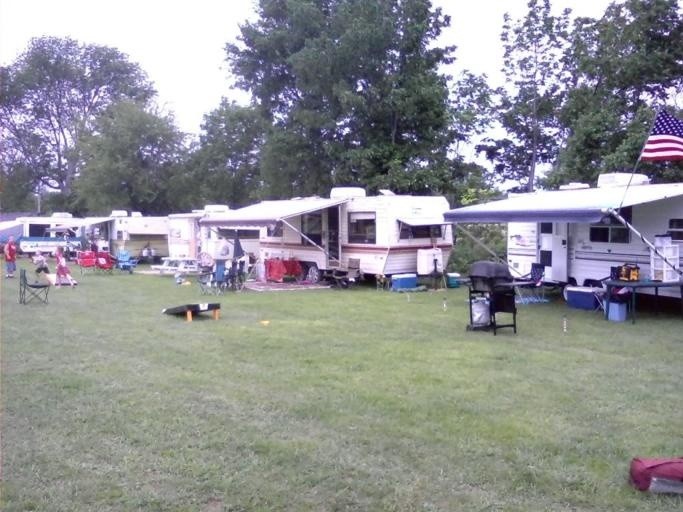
[233,227,245,269]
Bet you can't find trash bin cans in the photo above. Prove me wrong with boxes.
[602,292,627,322]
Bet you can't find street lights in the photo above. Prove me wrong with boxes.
[37,176,49,213]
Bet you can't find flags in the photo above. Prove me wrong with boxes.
[639,109,683,164]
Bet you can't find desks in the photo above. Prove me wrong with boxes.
[159,258,200,277]
[264,258,303,282]
[604,279,683,323]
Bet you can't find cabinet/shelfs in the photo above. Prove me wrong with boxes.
[649,245,680,283]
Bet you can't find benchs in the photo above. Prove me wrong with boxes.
[150,265,199,277]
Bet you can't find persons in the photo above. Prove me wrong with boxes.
[54,245,74,288]
[4,236,17,277]
[31,250,50,283]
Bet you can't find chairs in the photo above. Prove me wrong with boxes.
[76,250,114,274]
[343,258,361,289]
[513,263,545,304]
[19,269,51,305]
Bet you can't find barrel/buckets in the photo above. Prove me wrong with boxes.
[447,272,461,288]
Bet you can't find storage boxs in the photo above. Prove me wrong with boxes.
[392,273,417,292]
[446,273,461,288]
[603,298,627,321]
[566,286,599,311]
